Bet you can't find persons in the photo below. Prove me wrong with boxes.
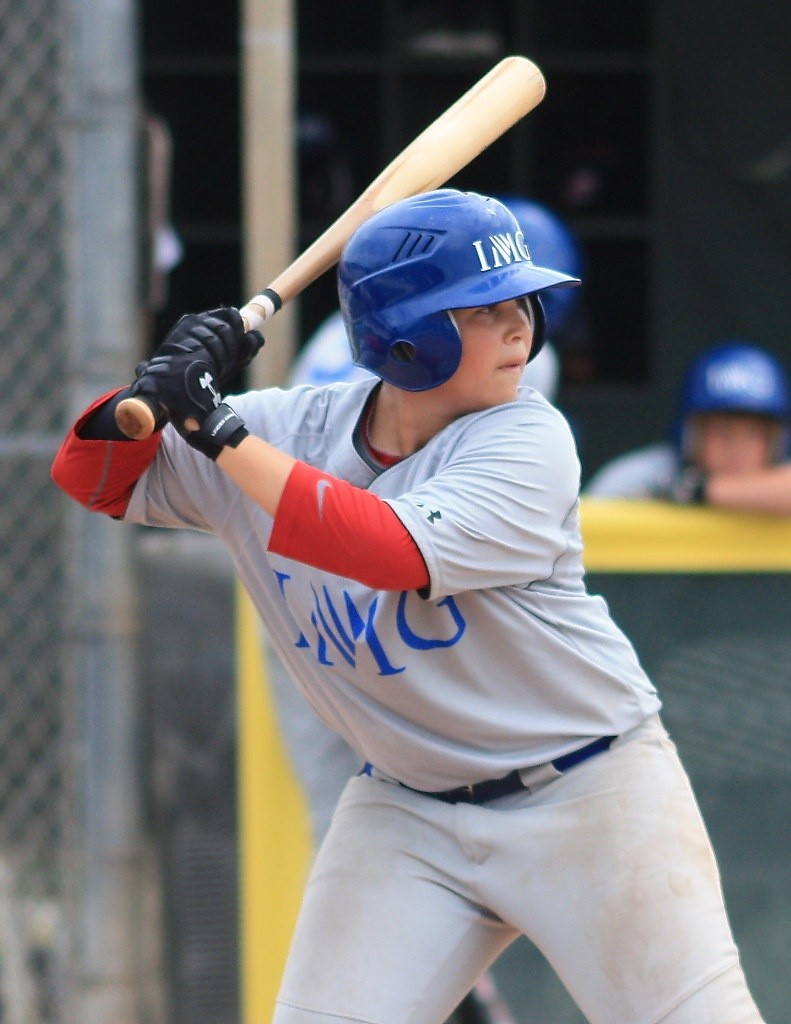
[287,198,579,406]
[51,188,765,1024]
[581,343,791,516]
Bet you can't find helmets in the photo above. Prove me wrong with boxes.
[495,195,577,332]
[335,189,580,391]
[671,341,791,466]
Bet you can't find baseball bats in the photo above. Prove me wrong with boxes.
[115,55,547,441]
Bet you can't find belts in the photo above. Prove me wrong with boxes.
[400,734,615,805]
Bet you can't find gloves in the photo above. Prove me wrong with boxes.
[127,343,246,461]
[130,307,268,406]
